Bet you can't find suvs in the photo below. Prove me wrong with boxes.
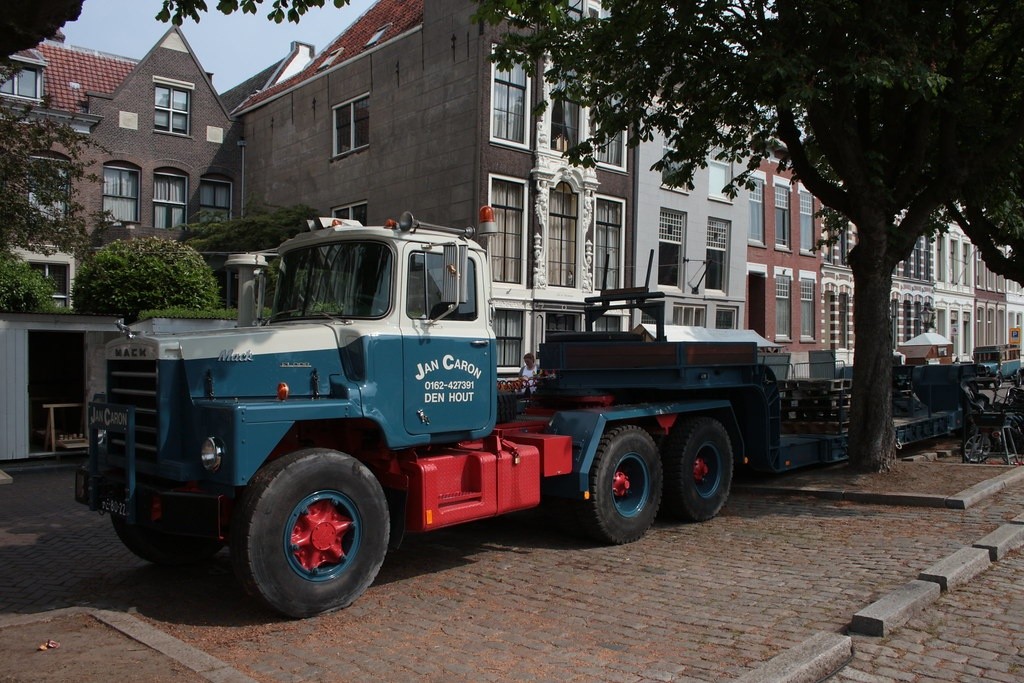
[972,344,1022,389]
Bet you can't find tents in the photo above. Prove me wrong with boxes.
[632,323,788,354]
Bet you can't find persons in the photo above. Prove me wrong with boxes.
[519,353,541,395]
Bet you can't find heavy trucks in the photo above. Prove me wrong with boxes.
[75,207,978,620]
